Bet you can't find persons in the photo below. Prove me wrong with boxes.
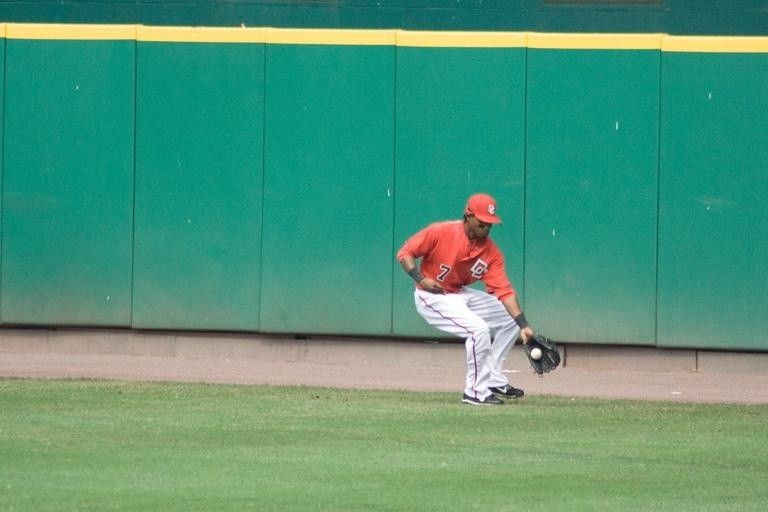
[396,192,563,408]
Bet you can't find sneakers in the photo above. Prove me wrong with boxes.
[462,392,504,406]
[488,383,524,398]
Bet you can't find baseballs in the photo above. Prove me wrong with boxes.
[530,348,543,360]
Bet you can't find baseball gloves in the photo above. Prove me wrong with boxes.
[524,333,561,376]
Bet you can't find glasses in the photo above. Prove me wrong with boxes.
[469,213,492,229]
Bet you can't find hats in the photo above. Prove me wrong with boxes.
[468,194,501,224]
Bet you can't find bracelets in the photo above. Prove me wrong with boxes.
[514,312,530,331]
[407,267,426,285]
